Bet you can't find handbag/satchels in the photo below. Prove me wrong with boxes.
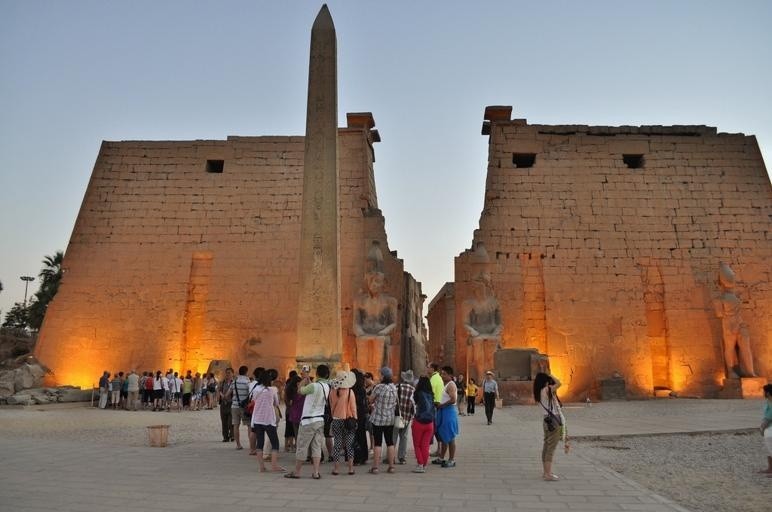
[495,399,503,408]
[394,416,405,428]
[324,414,333,438]
[210,383,216,392]
[239,397,255,418]
[345,418,357,429]
[161,388,165,395]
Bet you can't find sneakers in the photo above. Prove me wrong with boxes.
[487,421,492,425]
[98,403,217,411]
[222,437,455,479]
[459,412,474,416]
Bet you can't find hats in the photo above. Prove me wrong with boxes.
[486,371,495,377]
[379,367,392,377]
[401,370,414,382]
[302,365,310,372]
[332,371,357,390]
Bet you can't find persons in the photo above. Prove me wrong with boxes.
[758,385,772,478]
[216,362,479,478]
[712,260,757,381]
[534,357,564,481]
[480,370,500,424]
[353,271,398,381]
[462,270,504,386]
[97,368,216,411]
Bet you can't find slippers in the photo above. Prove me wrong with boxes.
[544,472,559,481]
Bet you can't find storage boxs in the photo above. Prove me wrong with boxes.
[146,425,168,448]
